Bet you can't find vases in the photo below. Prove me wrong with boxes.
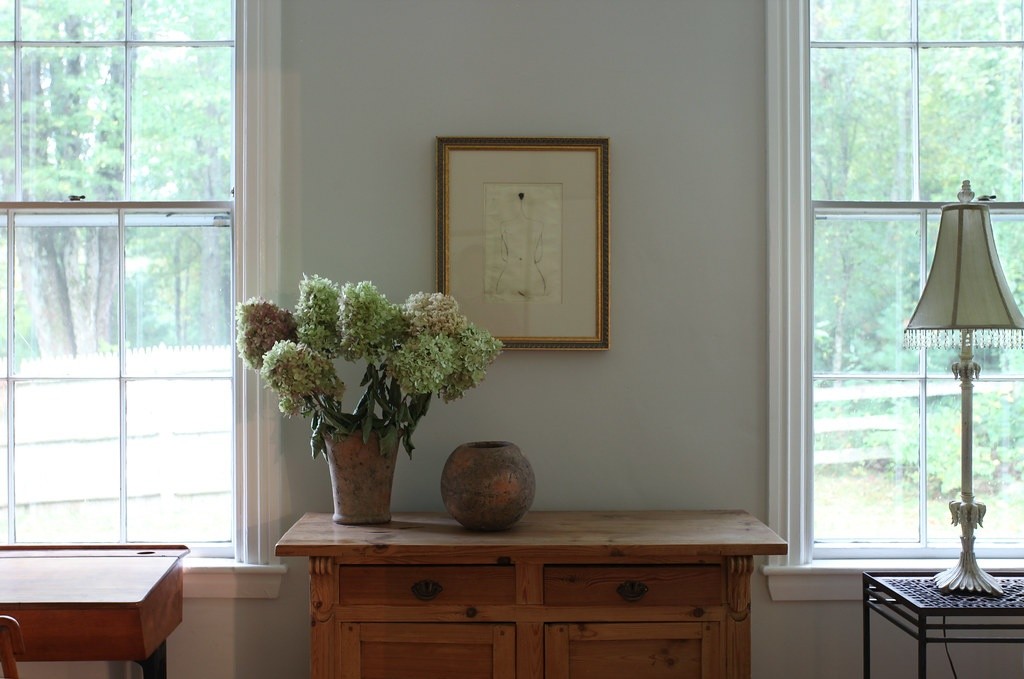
[321,428,403,524]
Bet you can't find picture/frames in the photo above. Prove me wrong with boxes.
[435,136,611,351]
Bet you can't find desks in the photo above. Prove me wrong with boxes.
[862,572,1024,679]
[0,545,191,679]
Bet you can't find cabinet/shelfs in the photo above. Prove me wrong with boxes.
[274,509,788,679]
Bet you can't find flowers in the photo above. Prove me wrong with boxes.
[233,272,506,460]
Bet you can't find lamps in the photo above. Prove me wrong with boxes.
[902,180,1024,596]
